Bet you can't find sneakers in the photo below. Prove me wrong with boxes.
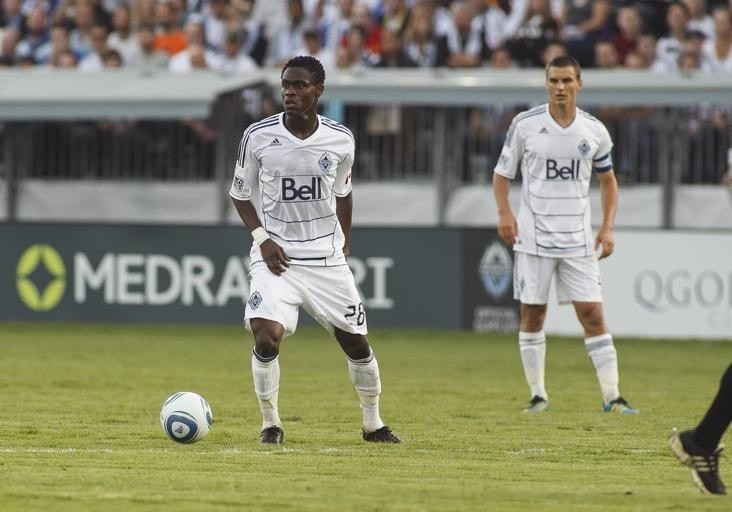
[668,427,729,497]
[600,396,641,416]
[521,394,551,414]
[361,425,403,444]
[259,425,284,445]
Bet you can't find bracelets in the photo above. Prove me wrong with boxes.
[248,227,272,246]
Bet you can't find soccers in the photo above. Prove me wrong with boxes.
[159,392,212,444]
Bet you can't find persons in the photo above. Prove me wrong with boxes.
[226,54,404,446]
[664,362,731,497]
[0,0,731,187]
[490,54,643,419]
